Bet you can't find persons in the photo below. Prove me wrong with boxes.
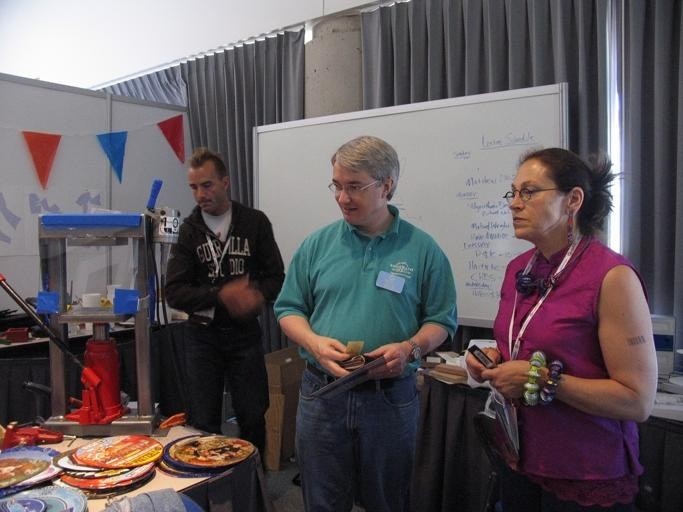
[468,147,658,508]
[273,136,457,511]
[165,146,286,477]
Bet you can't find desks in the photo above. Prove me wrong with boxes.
[2,409,258,512]
[417,356,682,509]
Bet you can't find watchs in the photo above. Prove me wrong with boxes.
[403,336,421,362]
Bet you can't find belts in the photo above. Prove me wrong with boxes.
[304,366,398,390]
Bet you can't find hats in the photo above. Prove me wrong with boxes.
[328,180,382,193]
[503,187,558,203]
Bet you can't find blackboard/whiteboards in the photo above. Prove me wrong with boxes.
[252,82,570,329]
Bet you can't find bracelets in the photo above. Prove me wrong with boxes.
[522,349,547,408]
[540,359,563,407]
